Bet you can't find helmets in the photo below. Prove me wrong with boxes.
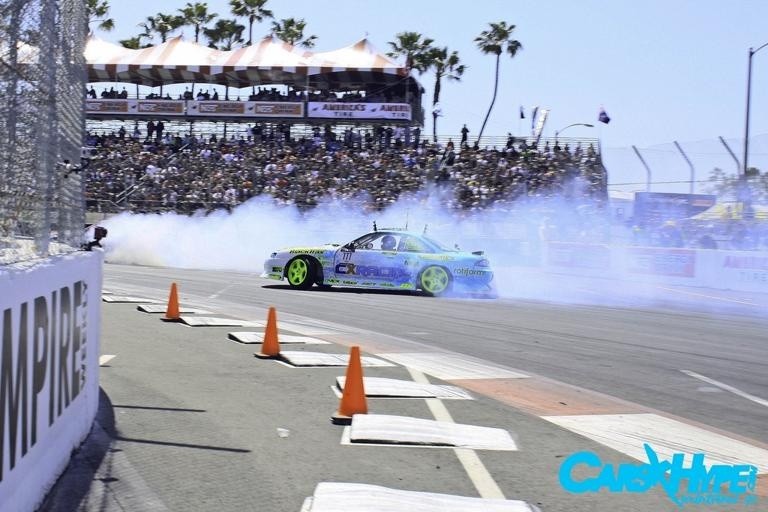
[379,235,397,250]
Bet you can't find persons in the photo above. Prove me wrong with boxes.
[611,197,768,253]
[84,83,409,102]
[77,113,610,237]
[364,235,397,252]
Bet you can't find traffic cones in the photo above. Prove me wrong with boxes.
[329,346,369,421]
[160,282,182,322]
[253,308,289,361]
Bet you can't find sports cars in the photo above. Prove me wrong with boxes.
[258,227,497,304]
